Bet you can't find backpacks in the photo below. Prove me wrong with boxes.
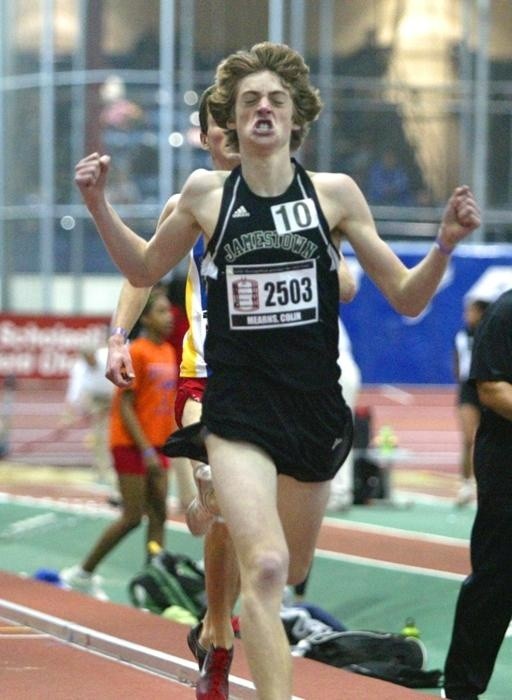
[127,552,207,622]
[279,603,445,689]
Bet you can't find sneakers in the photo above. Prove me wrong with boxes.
[187,622,234,699]
[60,566,109,603]
[186,464,220,537]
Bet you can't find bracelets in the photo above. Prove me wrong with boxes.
[435,239,453,253]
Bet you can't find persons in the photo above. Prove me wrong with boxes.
[74,43,482,700]
[453,288,512,699]
[68,73,416,700]
